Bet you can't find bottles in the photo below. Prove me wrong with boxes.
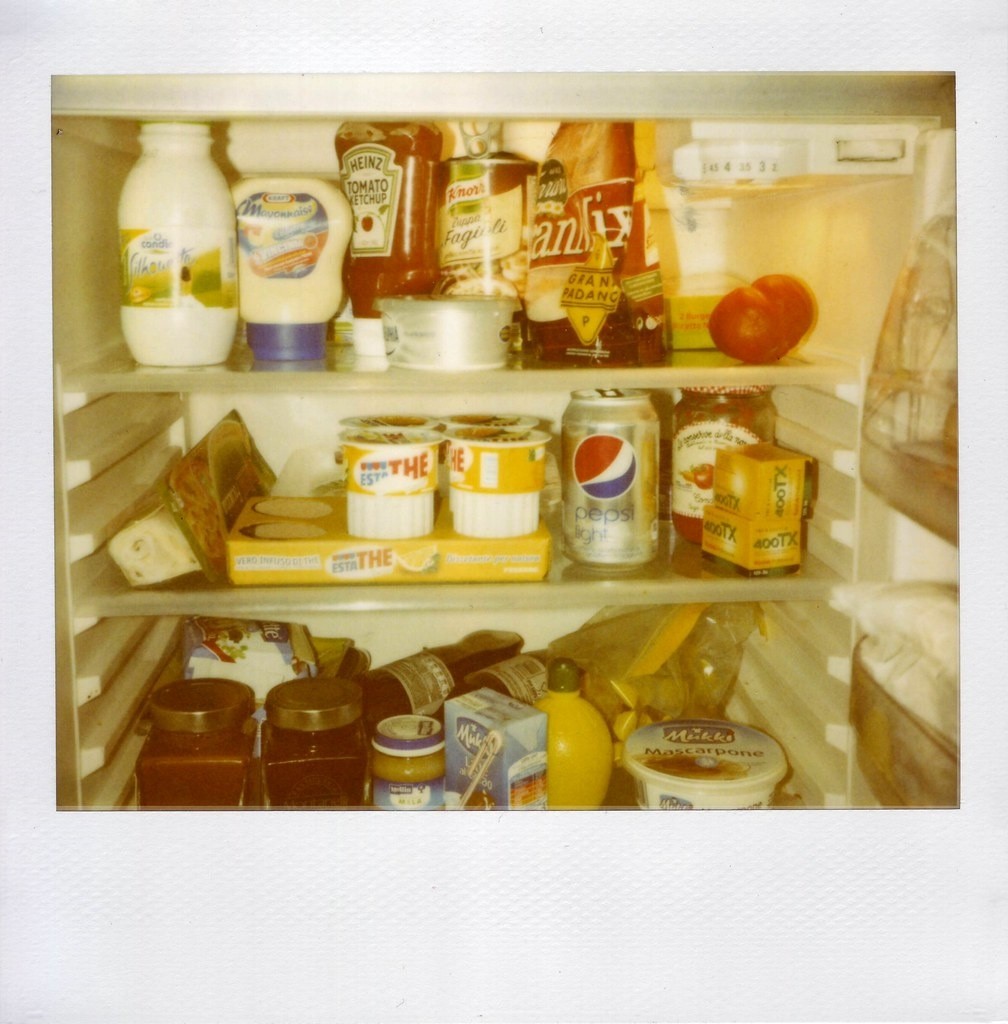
[436,647,553,709]
[116,122,238,365]
[342,629,525,731]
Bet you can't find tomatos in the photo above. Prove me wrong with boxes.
[708,274,814,363]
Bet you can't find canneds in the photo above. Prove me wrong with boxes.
[673,386,779,546]
[370,714,447,805]
[560,389,661,574]
